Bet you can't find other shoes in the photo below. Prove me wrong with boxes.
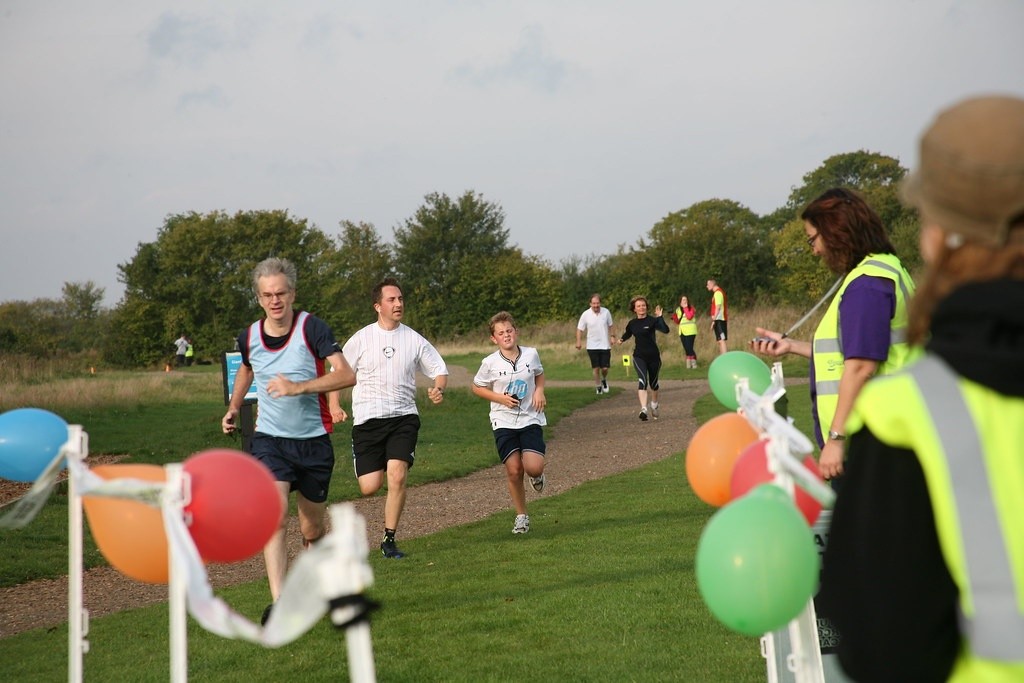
[261,603,273,625]
[692,359,697,368]
[687,359,691,368]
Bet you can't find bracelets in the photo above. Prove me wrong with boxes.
[712,320,715,322]
[611,335,615,338]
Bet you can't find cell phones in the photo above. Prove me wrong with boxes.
[510,393,520,404]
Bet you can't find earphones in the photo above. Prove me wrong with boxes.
[377,307,381,313]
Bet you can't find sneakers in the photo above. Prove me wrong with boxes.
[651,402,660,417]
[512,514,530,534]
[596,386,603,394]
[381,536,403,558]
[639,408,649,421]
[529,473,545,493]
[601,379,610,393]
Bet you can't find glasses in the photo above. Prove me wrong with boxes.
[807,232,820,247]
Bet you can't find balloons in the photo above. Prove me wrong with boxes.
[0,407,68,483]
[694,480,822,639]
[729,440,824,526]
[708,348,772,410]
[83,463,169,585]
[686,413,761,509]
[179,448,283,564]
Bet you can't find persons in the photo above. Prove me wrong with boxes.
[748,187,926,480]
[706,278,728,355]
[328,282,450,557]
[174,335,194,368]
[618,295,670,422]
[471,311,547,536]
[575,294,616,395]
[222,255,357,629]
[672,295,698,369]
[815,97,1024,683]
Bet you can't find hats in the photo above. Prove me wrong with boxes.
[903,95,1024,247]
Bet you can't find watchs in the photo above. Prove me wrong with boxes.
[829,430,847,441]
[437,387,444,397]
